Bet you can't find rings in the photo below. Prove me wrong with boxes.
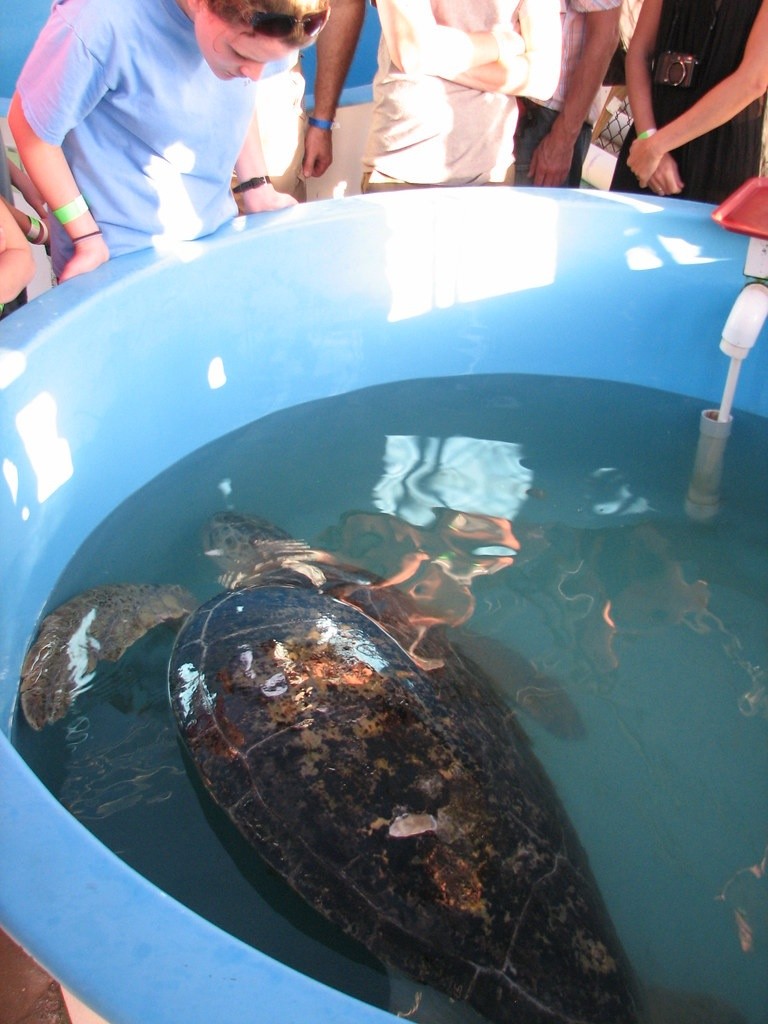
[656,185,662,192]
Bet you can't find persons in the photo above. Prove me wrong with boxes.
[0,0,767,327]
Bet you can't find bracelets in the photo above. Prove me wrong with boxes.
[52,195,89,225]
[308,116,337,131]
[38,220,49,247]
[72,232,102,245]
[27,215,40,244]
[635,129,659,141]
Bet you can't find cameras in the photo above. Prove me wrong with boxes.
[653,51,700,90]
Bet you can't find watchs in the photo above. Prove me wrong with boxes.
[232,176,270,195]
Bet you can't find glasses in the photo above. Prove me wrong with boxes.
[252,6,331,39]
